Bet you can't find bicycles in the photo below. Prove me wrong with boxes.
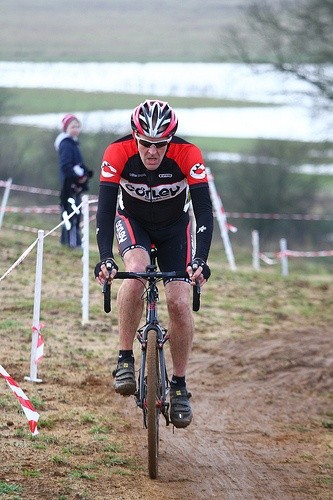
[102,247,201,479]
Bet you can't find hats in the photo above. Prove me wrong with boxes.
[62,115,80,132]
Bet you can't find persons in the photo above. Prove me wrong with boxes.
[94,99,213,428]
[50,114,95,249]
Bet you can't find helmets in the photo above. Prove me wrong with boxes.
[131,100,178,143]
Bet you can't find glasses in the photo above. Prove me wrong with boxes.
[135,133,172,148]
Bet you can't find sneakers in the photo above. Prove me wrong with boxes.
[112,356,136,393]
[170,382,193,428]
